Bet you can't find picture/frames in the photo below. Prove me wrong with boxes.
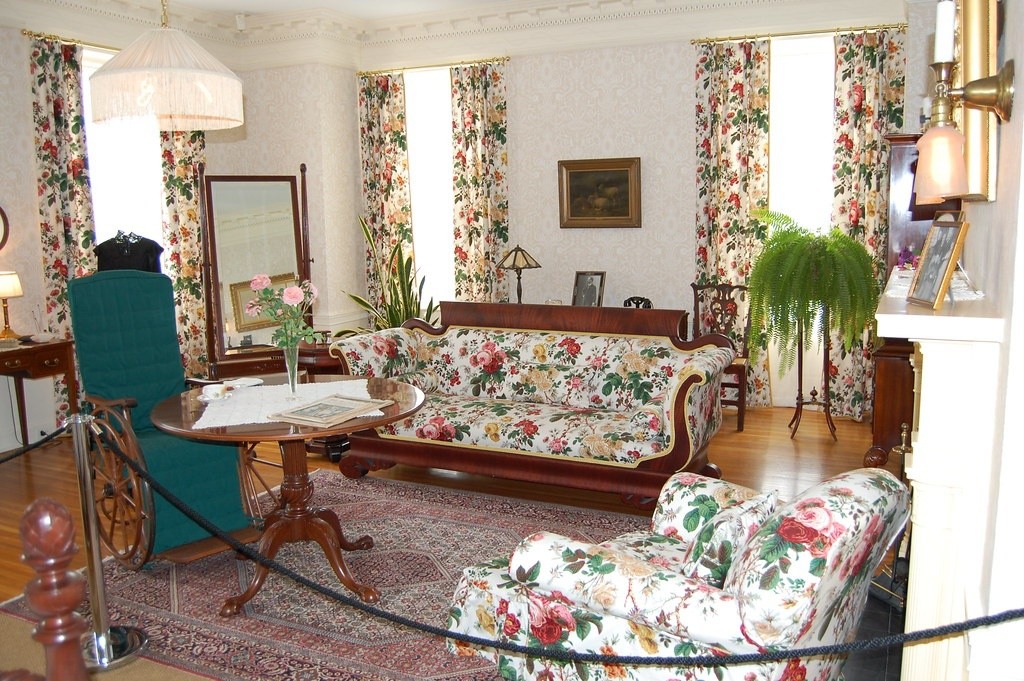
[232,270,299,333]
[961,0,998,202]
[572,271,606,307]
[935,211,965,222]
[556,158,641,228]
[907,221,969,308]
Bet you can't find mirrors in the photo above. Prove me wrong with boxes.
[205,175,307,361]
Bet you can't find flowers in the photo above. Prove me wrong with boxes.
[244,273,320,393]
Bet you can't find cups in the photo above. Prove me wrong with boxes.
[202,384,226,400]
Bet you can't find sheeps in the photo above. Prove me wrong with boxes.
[588,196,611,212]
[599,184,621,208]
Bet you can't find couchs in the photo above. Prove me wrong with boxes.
[328,302,736,515]
[445,464,907,680]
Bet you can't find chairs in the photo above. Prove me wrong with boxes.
[689,282,756,432]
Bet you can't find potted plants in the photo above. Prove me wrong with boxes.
[741,208,881,379]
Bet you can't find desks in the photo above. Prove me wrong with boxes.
[151,374,426,617]
[0,331,77,446]
[874,264,1023,681]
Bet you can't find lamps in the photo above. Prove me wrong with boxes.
[0,270,23,340]
[496,244,542,304]
[914,54,1016,209]
[87,0,245,133]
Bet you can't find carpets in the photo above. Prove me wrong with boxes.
[1,444,658,680]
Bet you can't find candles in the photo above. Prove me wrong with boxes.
[934,2,957,64]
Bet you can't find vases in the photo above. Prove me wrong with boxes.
[284,345,301,401]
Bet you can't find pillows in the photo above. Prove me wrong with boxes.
[678,488,779,586]
[386,369,440,394]
[630,388,669,442]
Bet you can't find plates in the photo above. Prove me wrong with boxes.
[196,393,232,404]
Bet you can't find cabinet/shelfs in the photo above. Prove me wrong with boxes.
[866,130,923,471]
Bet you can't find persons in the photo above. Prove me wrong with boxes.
[582,277,597,306]
[916,233,947,301]
